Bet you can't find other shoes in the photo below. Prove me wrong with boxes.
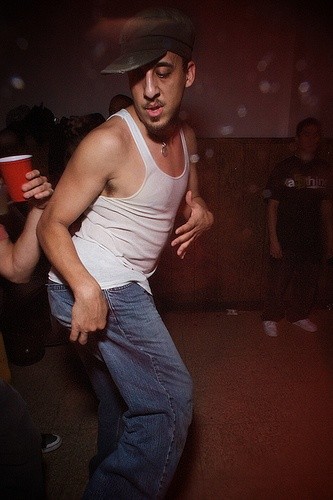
[40,433,62,453]
[264,321,279,337]
[293,319,318,332]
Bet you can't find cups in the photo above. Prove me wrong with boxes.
[0,155,36,202]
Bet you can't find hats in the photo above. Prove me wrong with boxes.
[100,5,196,73]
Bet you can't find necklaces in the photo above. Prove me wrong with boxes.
[154,124,178,157]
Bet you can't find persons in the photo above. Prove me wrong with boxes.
[36,17,213,500]
[262,118,333,337]
[0,95,133,500]
